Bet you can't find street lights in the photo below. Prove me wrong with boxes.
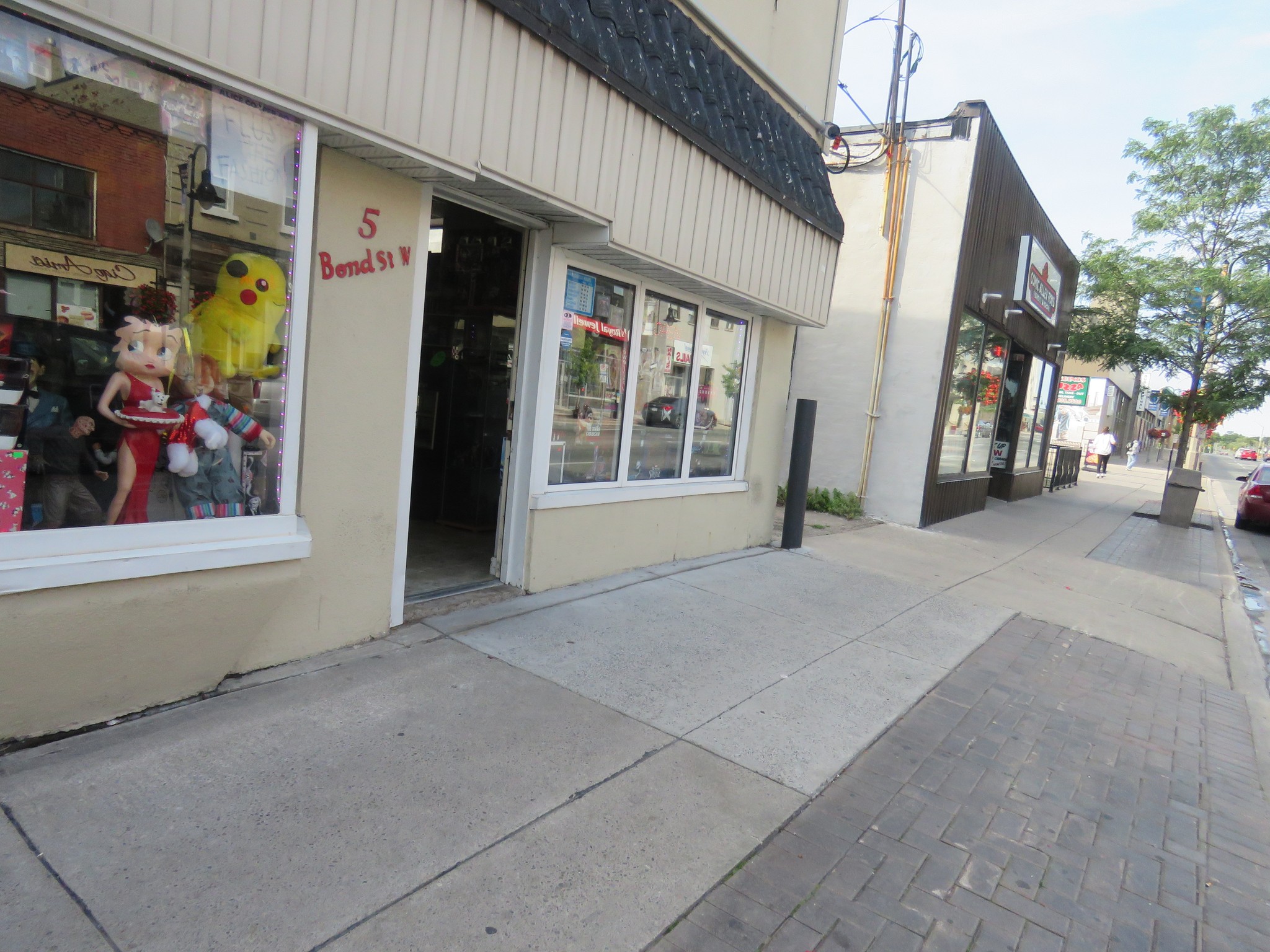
[179,140,225,338]
[1203,443,1213,453]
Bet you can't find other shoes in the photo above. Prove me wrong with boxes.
[1125,467,1132,471]
[1096,473,1107,478]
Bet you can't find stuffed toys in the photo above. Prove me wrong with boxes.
[183,252,287,378]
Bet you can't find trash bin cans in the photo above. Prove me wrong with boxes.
[1158,468,1201,529]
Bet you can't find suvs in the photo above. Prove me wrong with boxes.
[975,420,992,437]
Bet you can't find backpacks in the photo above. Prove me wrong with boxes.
[1125,439,1139,451]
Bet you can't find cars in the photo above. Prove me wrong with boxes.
[1239,450,1257,461]
[1035,423,1044,433]
[1233,463,1270,530]
[1234,448,1250,458]
[1263,447,1270,462]
[642,396,717,431]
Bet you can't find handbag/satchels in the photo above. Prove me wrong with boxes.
[1110,443,1118,452]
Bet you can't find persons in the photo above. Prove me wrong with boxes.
[1125,439,1142,471]
[161,355,277,519]
[1092,426,1116,478]
[581,404,593,419]
[24,354,118,530]
[95,284,184,524]
